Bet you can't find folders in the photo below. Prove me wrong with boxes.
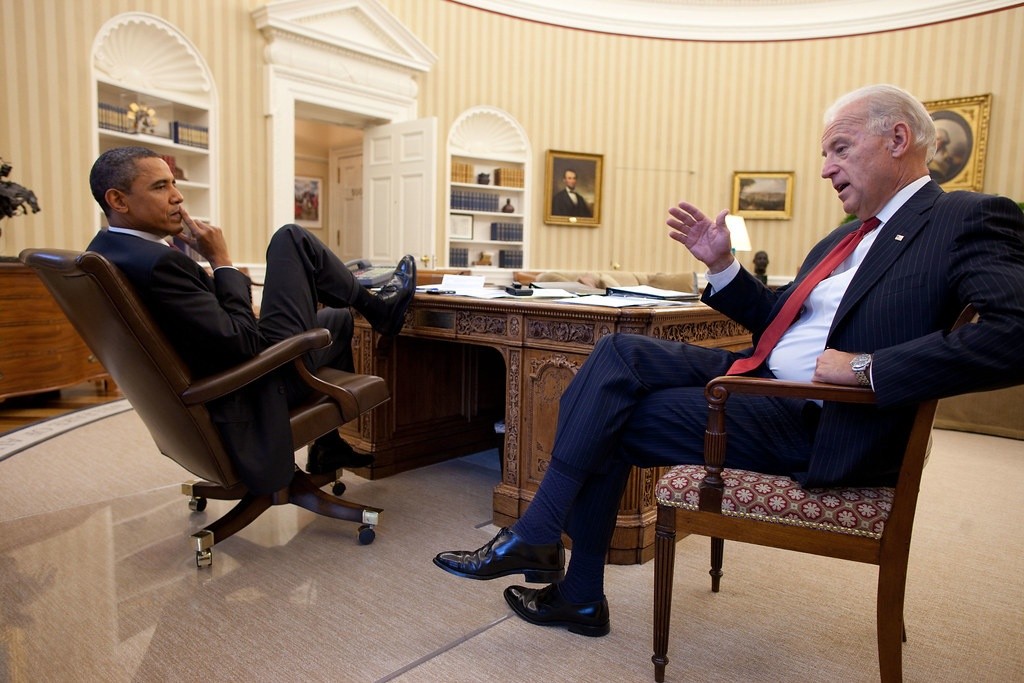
[606,284,700,300]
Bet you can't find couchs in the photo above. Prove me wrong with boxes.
[510,267,700,296]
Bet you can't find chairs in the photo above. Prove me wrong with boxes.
[651,300,976,682]
[18,247,393,569]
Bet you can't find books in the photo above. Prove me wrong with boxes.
[95,101,209,151]
[448,158,526,275]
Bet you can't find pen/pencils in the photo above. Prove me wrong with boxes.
[416,288,438,290]
[426,291,455,294]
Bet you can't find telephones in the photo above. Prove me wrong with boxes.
[343,258,397,287]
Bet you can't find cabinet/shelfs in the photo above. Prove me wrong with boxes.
[91,10,218,267]
[448,105,534,269]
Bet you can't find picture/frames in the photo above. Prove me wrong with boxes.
[731,170,796,219]
[544,149,605,228]
[292,175,324,229]
[917,92,995,195]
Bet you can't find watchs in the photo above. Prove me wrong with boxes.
[847,352,872,387]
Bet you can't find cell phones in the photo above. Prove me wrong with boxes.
[426,290,456,295]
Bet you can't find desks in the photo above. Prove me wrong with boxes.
[306,284,753,566]
[1,256,118,402]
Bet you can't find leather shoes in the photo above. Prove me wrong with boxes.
[377,254,416,337]
[504,583,611,637]
[306,439,376,473]
[433,527,565,583]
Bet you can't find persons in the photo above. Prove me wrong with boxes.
[83,145,417,497]
[551,167,593,219]
[429,83,1024,639]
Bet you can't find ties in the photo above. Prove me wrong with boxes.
[725,217,883,376]
